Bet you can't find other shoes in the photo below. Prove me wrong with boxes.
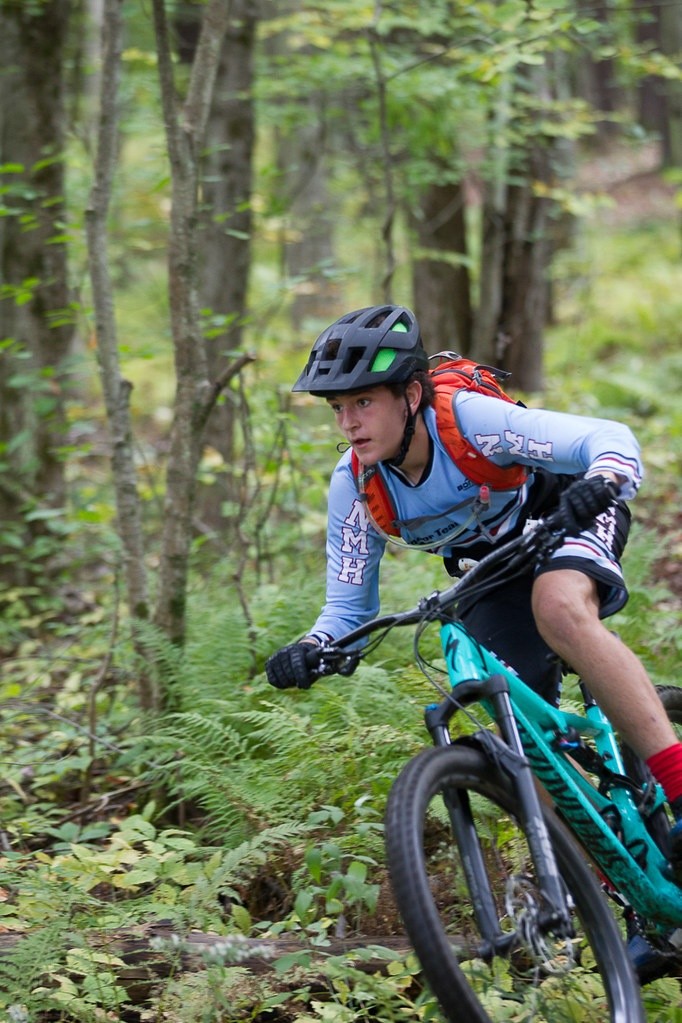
[620,905,671,986]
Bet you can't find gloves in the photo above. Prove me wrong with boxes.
[559,473,616,538]
[265,642,321,689]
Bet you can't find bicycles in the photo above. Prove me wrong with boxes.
[262,477,681,1023]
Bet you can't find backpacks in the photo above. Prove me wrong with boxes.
[351,351,528,538]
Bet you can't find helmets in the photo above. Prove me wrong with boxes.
[290,306,429,392]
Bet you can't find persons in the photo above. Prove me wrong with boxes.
[266,306,682,872]
